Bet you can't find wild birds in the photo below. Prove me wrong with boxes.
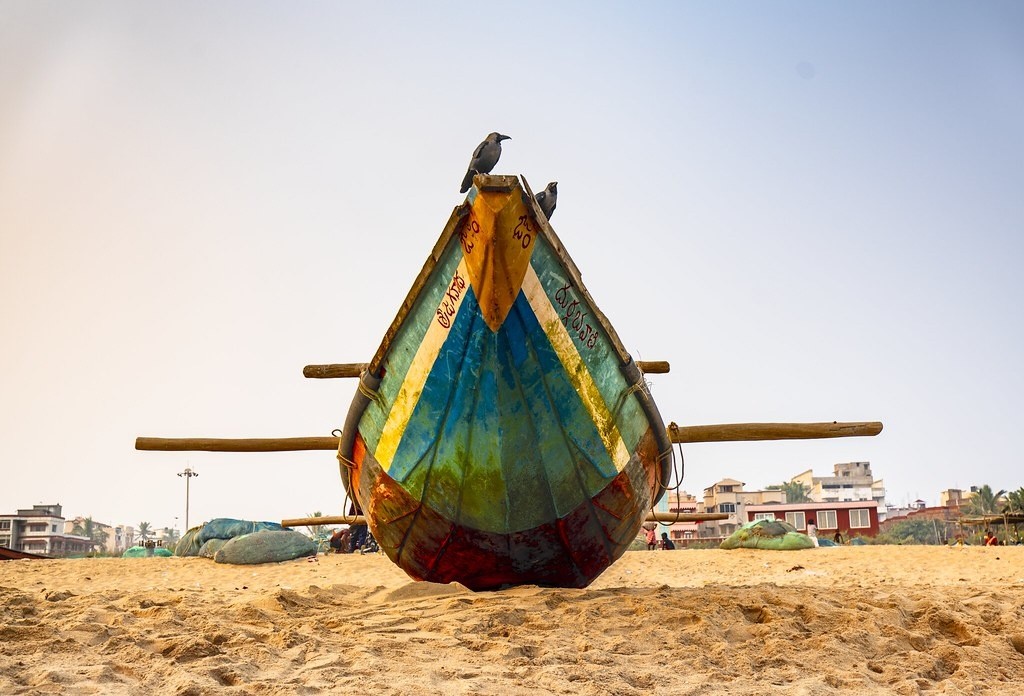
[460,131,512,195]
[534,182,559,223]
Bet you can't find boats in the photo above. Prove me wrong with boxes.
[136,174,883,591]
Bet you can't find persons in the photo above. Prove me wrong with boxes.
[347,502,367,555]
[833,528,844,544]
[984,532,998,546]
[348,531,380,553]
[642,524,657,551]
[806,519,819,548]
[329,527,353,554]
[660,532,675,551]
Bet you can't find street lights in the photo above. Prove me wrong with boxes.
[177,468,199,533]
